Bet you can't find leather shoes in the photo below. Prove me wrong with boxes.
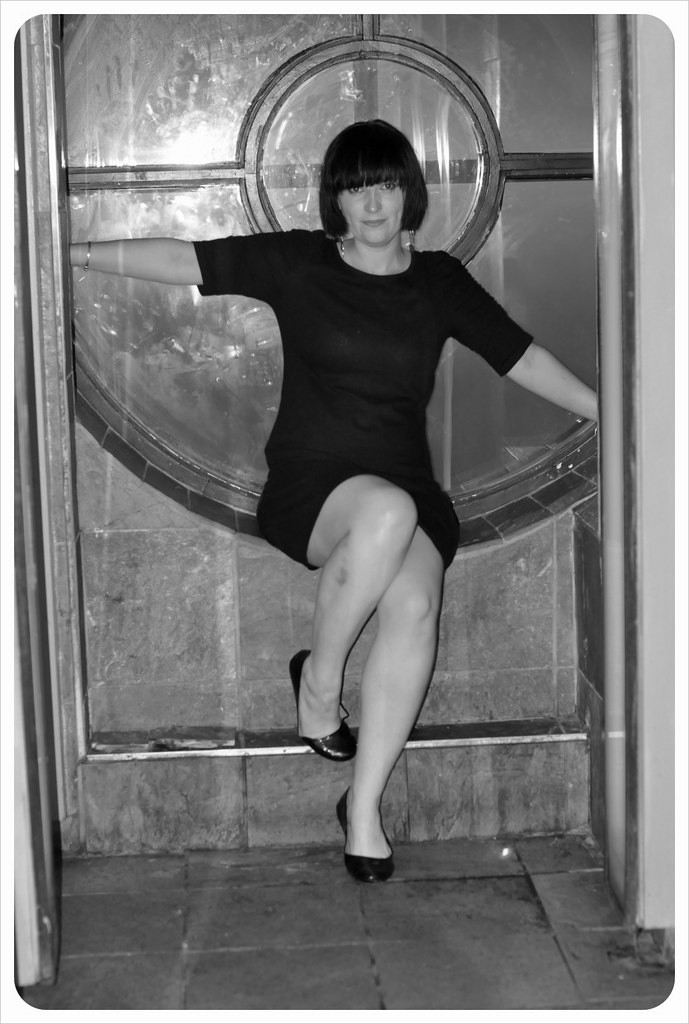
[289,650,357,762]
[337,785,395,882]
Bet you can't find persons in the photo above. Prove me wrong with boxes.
[68,119,602,886]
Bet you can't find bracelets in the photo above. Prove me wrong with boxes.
[84,241,91,273]
